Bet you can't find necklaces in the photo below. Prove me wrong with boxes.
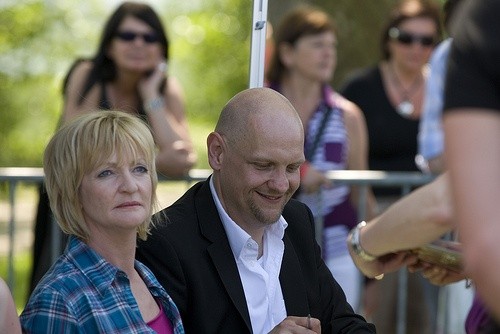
[387,62,426,118]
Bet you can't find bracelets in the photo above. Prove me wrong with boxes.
[351,221,379,263]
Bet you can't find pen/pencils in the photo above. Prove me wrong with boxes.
[307,314,313,330]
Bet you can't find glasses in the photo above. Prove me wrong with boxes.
[112,30,163,43]
[389,27,439,48]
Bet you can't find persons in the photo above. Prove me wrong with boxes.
[131,87,378,333]
[347,168,476,290]
[0,277,23,334]
[334,0,454,334]
[18,110,188,334]
[25,1,197,315]
[265,5,375,323]
[417,0,500,334]
